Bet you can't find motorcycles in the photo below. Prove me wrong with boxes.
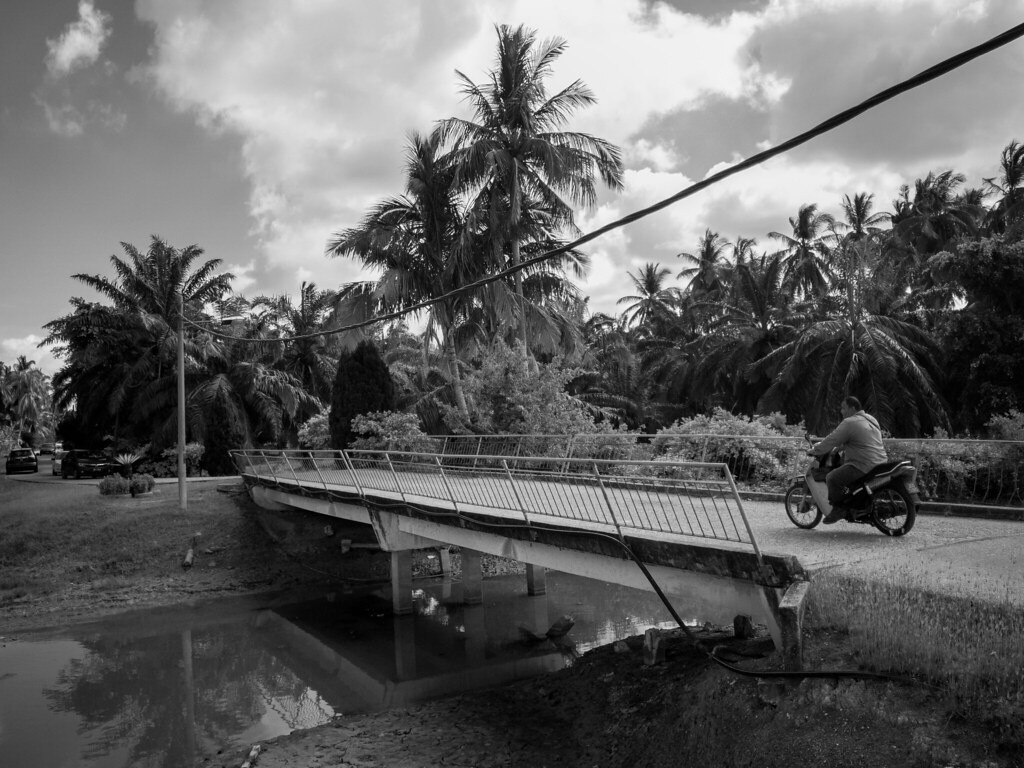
[784,432,922,537]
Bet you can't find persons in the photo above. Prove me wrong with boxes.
[807,396,887,524]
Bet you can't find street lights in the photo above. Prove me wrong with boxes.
[177,316,247,510]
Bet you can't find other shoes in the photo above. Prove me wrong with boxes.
[823,506,848,524]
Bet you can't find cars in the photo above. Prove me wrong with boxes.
[61,449,112,480]
[53,440,64,454]
[40,442,56,456]
[51,450,69,476]
[4,448,40,475]
